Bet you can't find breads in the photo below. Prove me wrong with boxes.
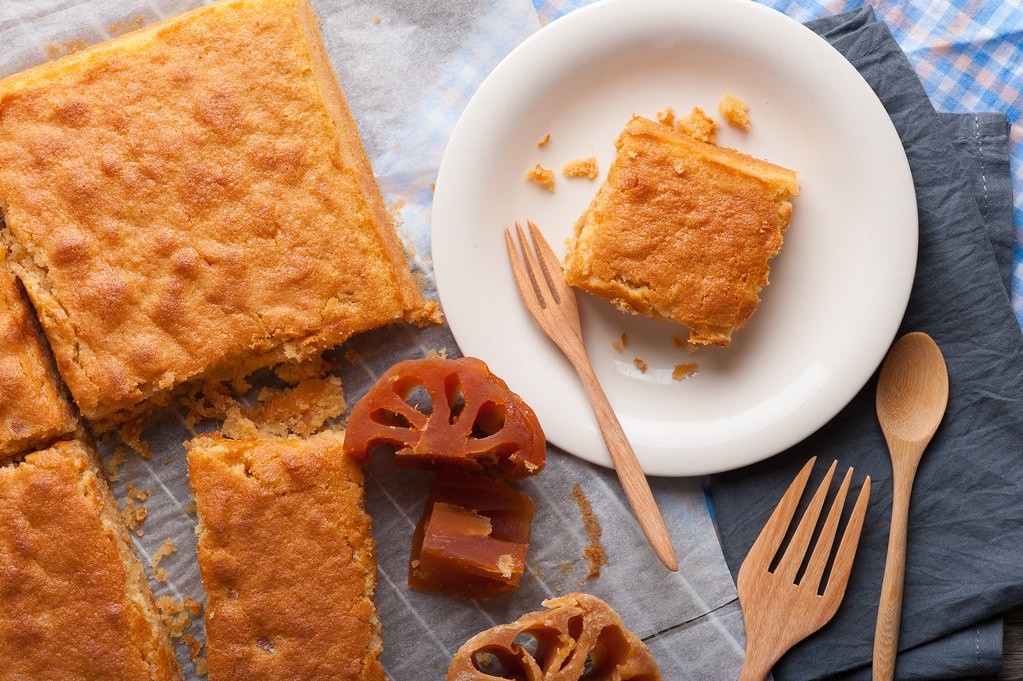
[0,0,443,681]
[559,114,800,346]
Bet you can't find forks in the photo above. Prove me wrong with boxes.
[503,217,678,572]
[737,457,871,681]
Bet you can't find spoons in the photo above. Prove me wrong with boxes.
[872,331,949,681]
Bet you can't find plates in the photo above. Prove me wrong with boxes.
[431,0,920,475]
